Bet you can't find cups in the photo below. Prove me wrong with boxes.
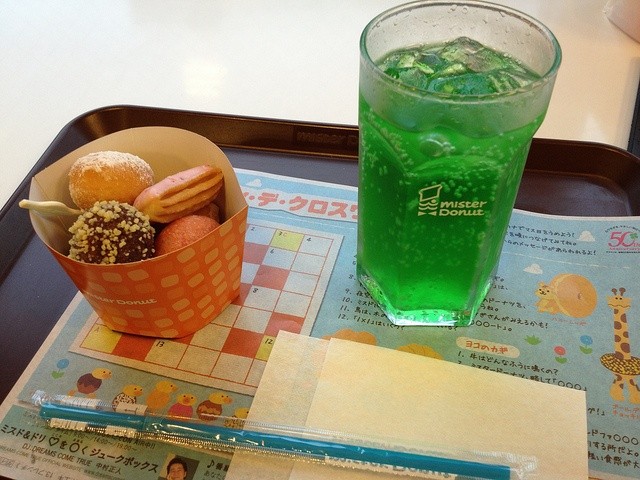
[357,0,562,328]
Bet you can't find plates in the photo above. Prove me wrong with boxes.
[1,102,640,480]
